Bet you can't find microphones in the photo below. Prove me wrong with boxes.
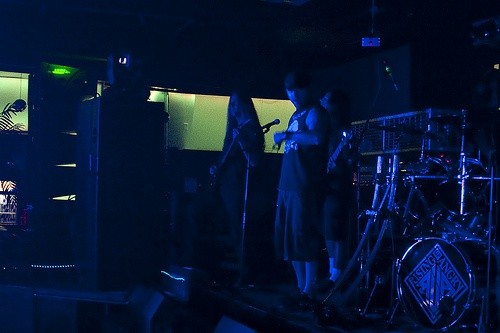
[387,66,399,92]
[262,119,280,129]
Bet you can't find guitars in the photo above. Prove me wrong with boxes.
[326,128,354,174]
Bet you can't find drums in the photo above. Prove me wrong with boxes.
[414,156,447,189]
[452,157,486,195]
[393,237,500,333]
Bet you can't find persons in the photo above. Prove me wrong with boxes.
[209,92,271,279]
[275,70,351,311]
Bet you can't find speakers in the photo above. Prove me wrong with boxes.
[75,97,167,291]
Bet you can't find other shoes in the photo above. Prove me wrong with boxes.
[278,288,316,309]
